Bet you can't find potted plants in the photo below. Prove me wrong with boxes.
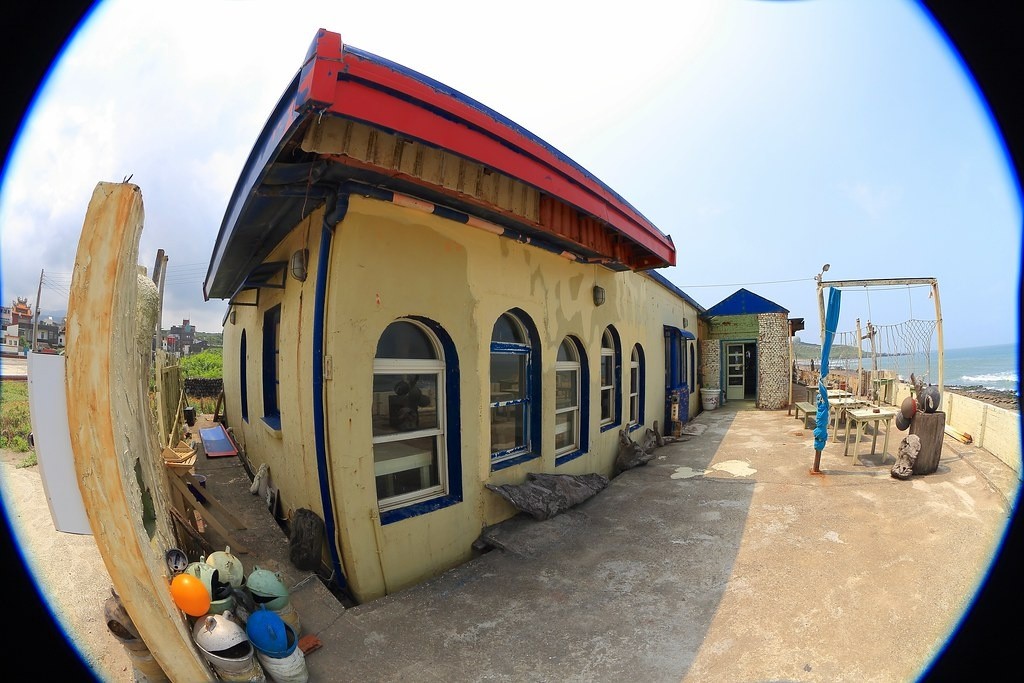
[699,382,721,410]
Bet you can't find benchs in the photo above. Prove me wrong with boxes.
[795,402,818,429]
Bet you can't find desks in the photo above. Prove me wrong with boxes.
[373,441,433,498]
[827,397,866,444]
[805,385,833,405]
[815,389,853,423]
[844,408,895,466]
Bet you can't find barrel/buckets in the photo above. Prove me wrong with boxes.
[187,474,206,505]
[701,388,721,410]
[247,620,309,683]
[213,657,265,683]
[277,604,302,638]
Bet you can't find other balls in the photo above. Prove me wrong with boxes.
[171,573,211,617]
[895,386,941,432]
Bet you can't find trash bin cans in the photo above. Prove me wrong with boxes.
[182,556,240,630]
[239,564,303,641]
[206,546,250,612]
[245,609,309,683]
[102,589,169,682]
[184,407,195,427]
[191,612,266,683]
